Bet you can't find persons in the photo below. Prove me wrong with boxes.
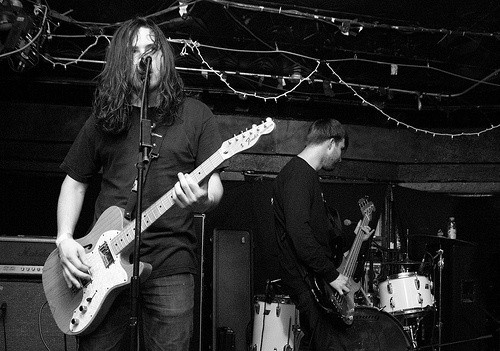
[54,17,223,351]
[272,118,376,351]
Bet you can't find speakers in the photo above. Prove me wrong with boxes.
[211,225,253,351]
[0,278,80,351]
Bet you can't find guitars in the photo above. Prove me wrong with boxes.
[309,195,376,325]
[42,117,276,336]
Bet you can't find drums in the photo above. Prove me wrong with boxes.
[377,271,437,319]
[301,305,415,351]
[251,294,300,351]
[363,262,382,296]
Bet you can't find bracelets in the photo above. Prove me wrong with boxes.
[56,233,73,248]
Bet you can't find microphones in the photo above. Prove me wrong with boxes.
[343,219,358,226]
[137,54,152,75]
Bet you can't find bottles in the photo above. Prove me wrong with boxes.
[448,217,456,240]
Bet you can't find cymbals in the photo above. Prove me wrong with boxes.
[409,233,476,247]
[383,260,423,265]
